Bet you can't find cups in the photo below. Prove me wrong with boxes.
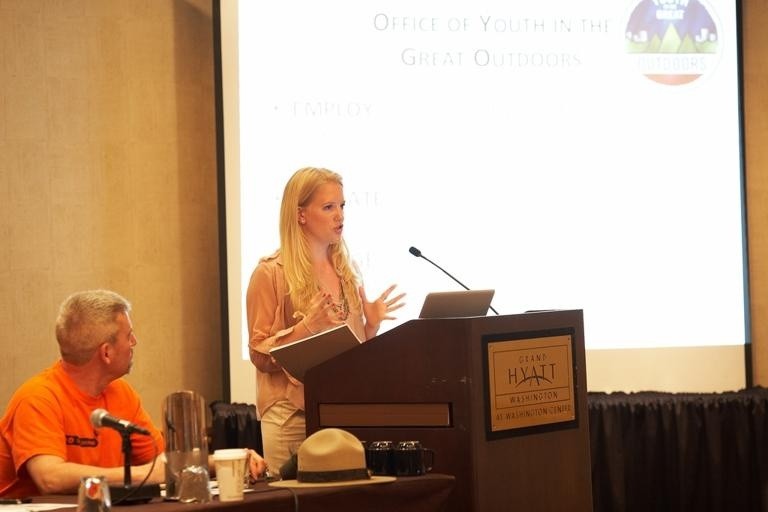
[396,439,435,476]
[367,440,398,476]
[213,447,249,501]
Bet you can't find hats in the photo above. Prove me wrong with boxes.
[267,428,398,490]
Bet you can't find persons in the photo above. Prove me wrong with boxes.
[246,168,407,474]
[0,289,270,503]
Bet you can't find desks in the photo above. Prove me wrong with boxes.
[1,473,458,512]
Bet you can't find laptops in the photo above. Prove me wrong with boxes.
[419,289,495,318]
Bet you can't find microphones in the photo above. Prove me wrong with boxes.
[90,408,151,435]
[409,246,499,315]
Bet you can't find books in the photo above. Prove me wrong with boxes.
[270,324,361,383]
